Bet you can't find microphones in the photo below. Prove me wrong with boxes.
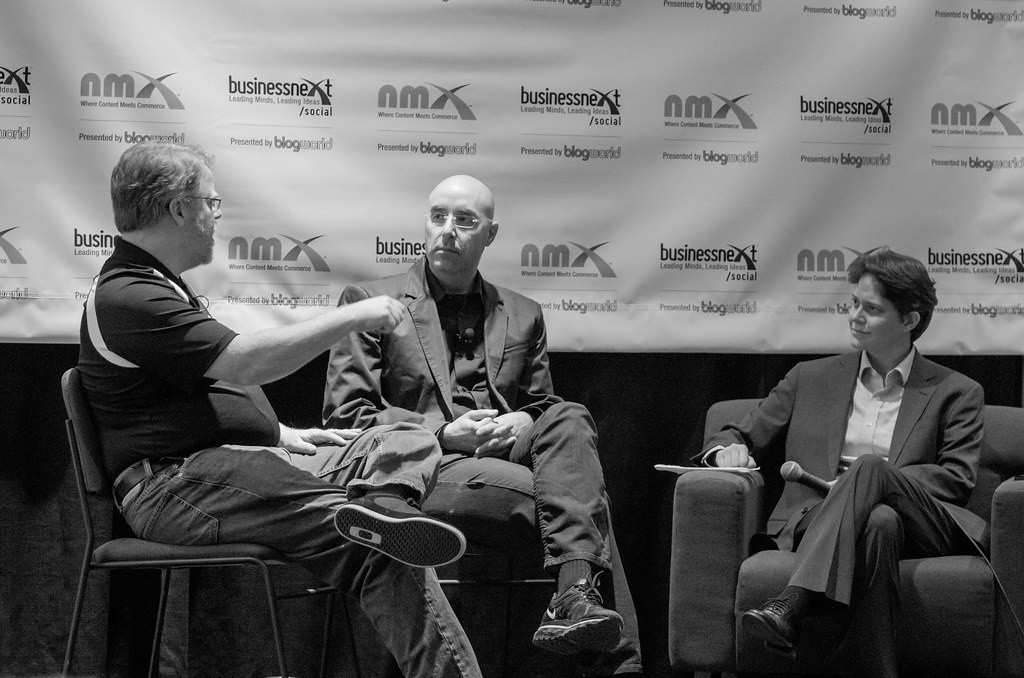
[465,329,475,344]
[780,462,830,496]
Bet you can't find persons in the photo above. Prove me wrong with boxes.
[77,141,483,678]
[323,174,642,678]
[689,252,984,678]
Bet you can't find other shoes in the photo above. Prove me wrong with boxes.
[334,487,467,569]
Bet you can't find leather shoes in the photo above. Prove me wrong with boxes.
[741,593,805,659]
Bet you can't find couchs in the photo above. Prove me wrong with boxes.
[668,399,1024,678]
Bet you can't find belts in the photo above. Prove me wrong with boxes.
[113,457,178,508]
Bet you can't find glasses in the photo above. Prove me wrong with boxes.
[185,195,222,210]
[423,208,494,230]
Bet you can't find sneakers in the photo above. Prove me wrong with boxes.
[531,571,625,661]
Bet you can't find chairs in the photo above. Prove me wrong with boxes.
[63,369,556,678]
